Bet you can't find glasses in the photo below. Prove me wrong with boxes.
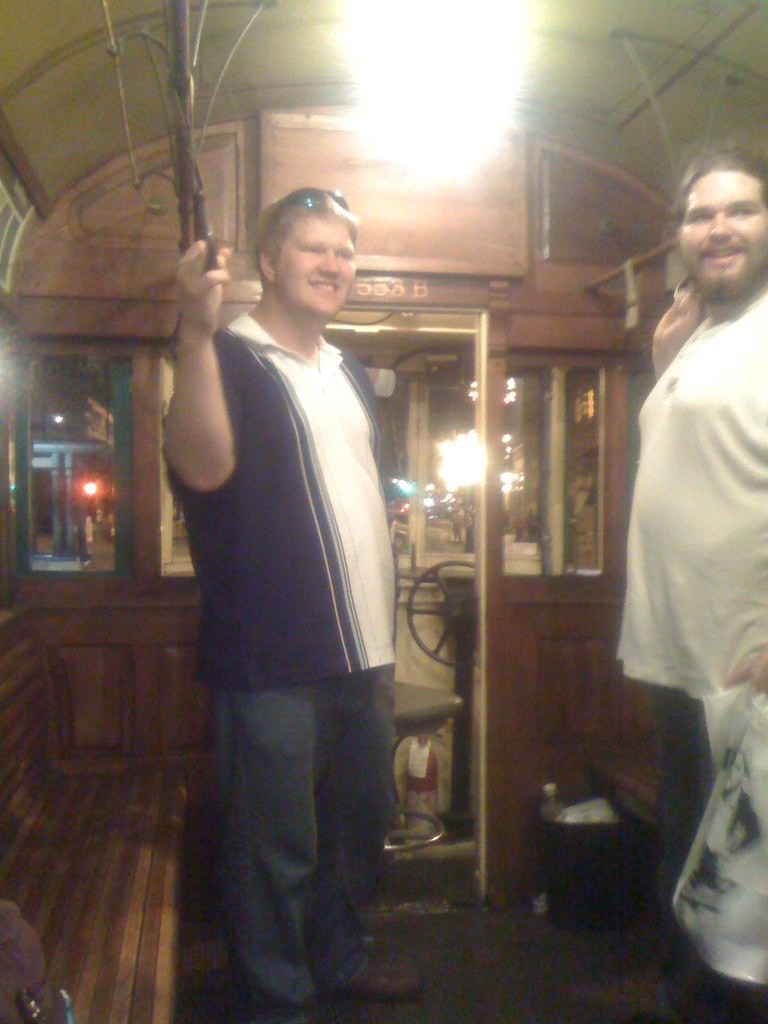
[257,188,349,244]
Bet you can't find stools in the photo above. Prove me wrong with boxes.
[373,678,465,853]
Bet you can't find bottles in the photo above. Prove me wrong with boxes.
[538,783,569,823]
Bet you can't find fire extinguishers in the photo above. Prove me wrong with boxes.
[392,728,445,844]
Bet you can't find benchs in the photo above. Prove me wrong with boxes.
[1,607,214,1024]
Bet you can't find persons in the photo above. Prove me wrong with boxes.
[169,182,423,1024]
[619,150,768,1023]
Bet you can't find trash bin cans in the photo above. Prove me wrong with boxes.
[538,787,642,933]
[503,533,516,562]
